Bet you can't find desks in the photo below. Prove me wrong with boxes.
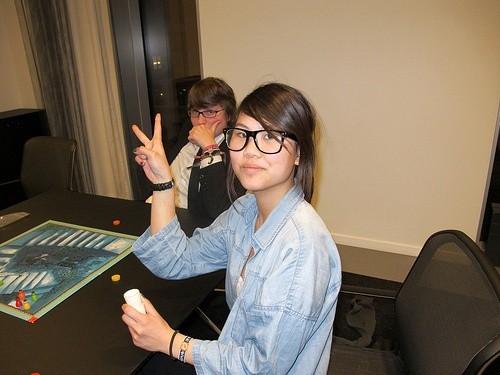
[0,188,226,375]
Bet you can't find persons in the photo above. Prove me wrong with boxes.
[122,83,343,375]
[145,77,247,221]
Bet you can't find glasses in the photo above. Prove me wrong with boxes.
[187,109,223,118]
[223,127,299,154]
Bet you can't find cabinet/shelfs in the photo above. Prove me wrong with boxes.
[0,108,52,211]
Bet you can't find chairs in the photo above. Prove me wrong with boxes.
[325,229,500,375]
[20,136,77,198]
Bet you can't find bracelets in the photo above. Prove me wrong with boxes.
[179,336,192,363]
[151,177,175,191]
[168,329,179,360]
[200,144,220,156]
